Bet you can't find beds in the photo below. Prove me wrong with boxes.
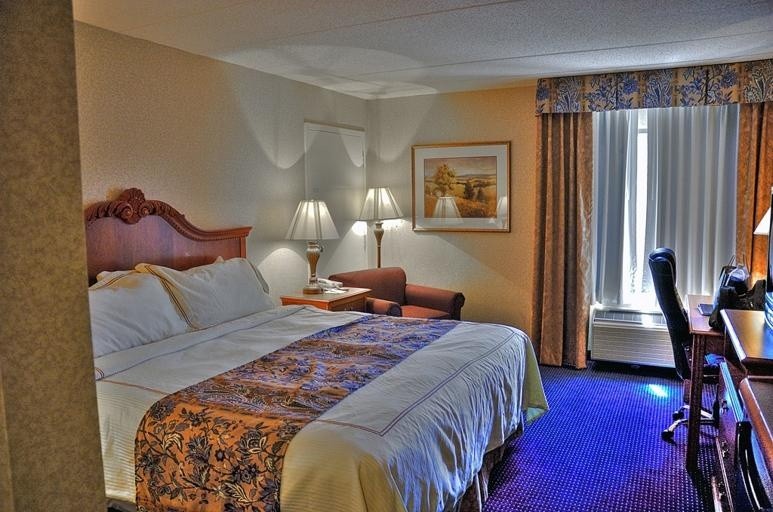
[84,187,550,511]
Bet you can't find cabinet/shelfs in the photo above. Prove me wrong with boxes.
[734,376,773,512]
[717,308,773,512]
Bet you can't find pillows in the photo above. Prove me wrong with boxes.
[134,257,277,331]
[88,269,195,359]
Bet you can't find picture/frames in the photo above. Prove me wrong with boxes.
[412,140,511,232]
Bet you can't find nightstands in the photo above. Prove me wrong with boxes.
[281,288,371,314]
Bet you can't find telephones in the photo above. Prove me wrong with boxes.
[318,278,343,288]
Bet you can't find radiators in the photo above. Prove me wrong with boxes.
[590,309,675,370]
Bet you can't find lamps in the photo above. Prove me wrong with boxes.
[754,206,773,237]
[283,198,340,294]
[355,188,406,263]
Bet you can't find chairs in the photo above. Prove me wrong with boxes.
[647,248,725,440]
[329,268,465,322]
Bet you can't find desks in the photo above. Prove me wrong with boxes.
[685,292,727,470]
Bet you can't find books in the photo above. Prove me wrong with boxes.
[696,303,712,316]
[324,289,346,295]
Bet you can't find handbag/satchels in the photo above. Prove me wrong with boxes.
[708,254,751,331]
